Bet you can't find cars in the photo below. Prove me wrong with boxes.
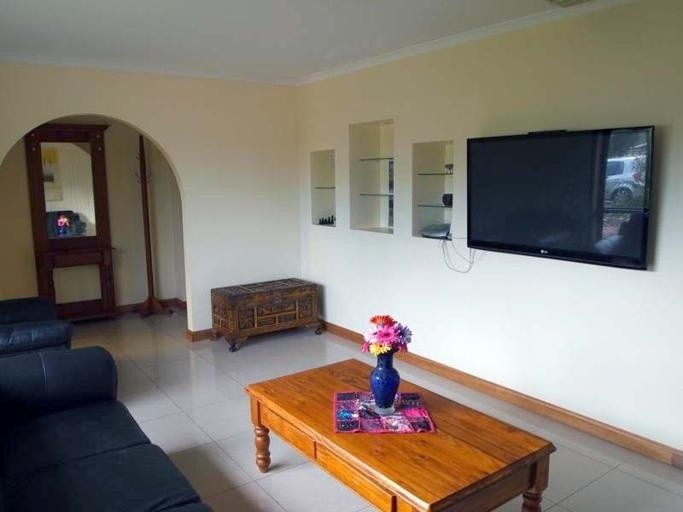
[605,155,646,201]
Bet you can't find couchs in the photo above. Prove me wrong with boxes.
[0,346,213,512]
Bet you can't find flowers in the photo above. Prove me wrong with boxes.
[58,215,71,227]
[361,314,413,355]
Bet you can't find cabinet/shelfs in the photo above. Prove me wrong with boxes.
[357,158,394,197]
[416,172,453,208]
[211,277,322,352]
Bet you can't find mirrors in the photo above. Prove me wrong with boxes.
[40,139,99,240]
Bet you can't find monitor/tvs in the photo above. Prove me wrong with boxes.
[467,125,654,269]
[46,211,74,237]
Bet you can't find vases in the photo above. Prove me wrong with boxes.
[60,227,67,235]
[369,351,401,415]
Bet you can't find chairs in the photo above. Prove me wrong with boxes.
[0,296,74,353]
